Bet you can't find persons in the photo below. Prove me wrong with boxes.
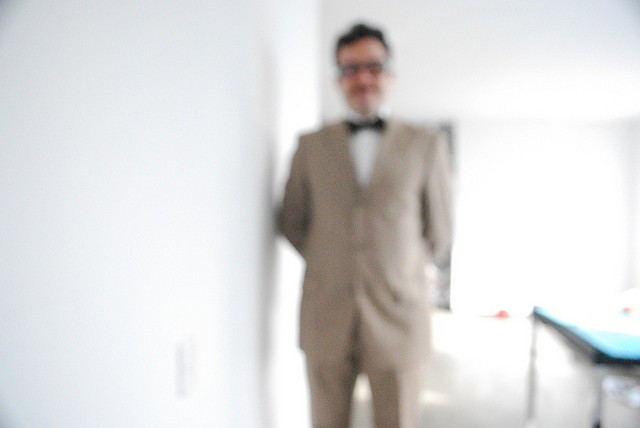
[278,21,455,428]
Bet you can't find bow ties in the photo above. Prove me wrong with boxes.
[345,118,387,139]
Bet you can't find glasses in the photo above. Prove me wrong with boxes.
[335,56,389,79]
